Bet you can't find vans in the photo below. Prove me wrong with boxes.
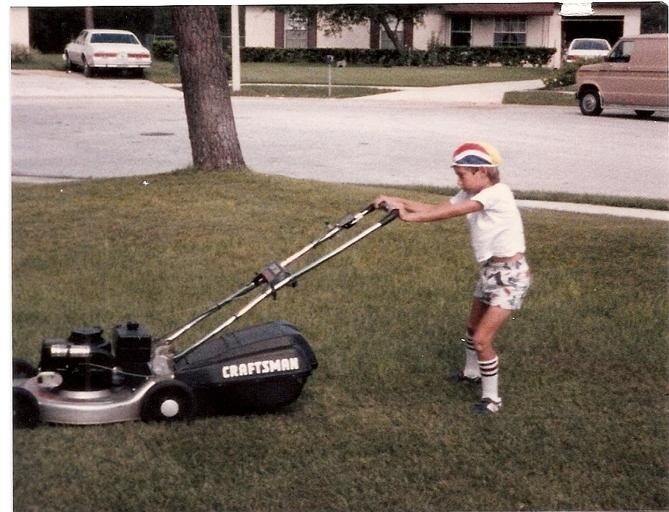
[573,34,668,119]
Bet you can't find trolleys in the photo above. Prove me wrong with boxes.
[13,194,405,433]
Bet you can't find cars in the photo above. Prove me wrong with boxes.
[562,36,619,70]
[60,29,153,81]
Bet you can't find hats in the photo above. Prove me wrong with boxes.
[448,139,504,170]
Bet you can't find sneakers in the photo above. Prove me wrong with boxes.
[473,395,504,416]
[442,370,482,388]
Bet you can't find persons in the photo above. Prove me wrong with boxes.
[372,141,533,415]
